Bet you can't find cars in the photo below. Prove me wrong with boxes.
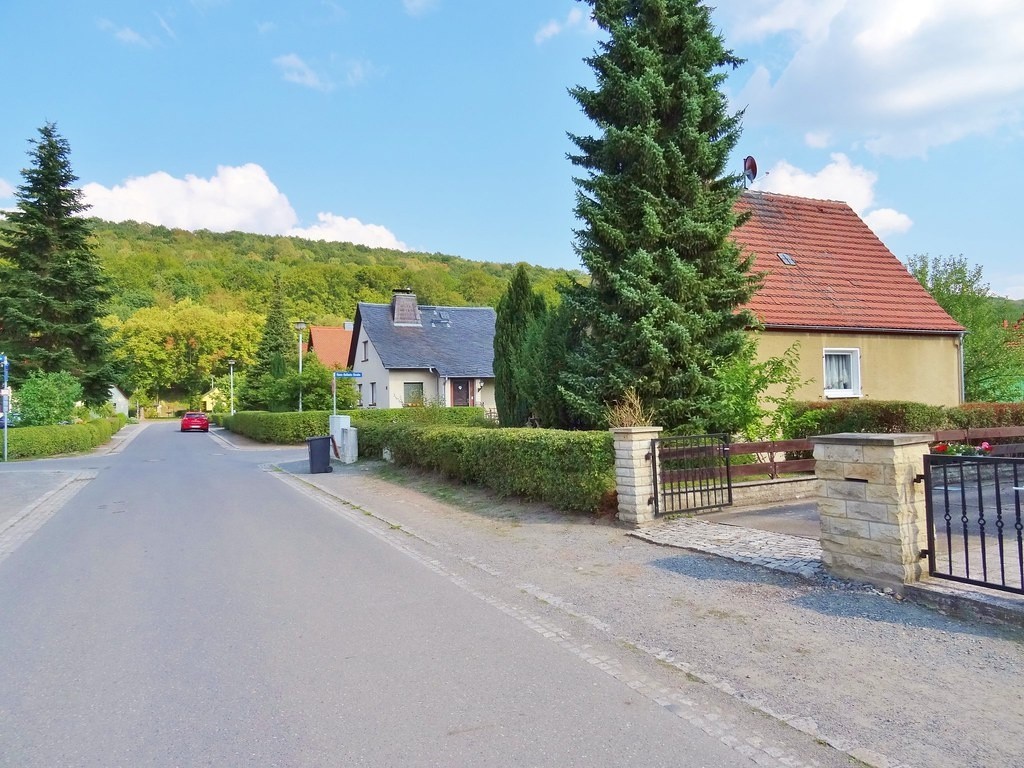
[180,412,210,432]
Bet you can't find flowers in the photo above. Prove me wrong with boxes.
[929,443,993,456]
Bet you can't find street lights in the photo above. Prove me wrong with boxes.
[228,358,237,414]
[291,316,309,411]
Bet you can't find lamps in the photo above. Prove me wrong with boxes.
[478,380,484,392]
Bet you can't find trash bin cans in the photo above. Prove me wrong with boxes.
[306,435,340,474]
